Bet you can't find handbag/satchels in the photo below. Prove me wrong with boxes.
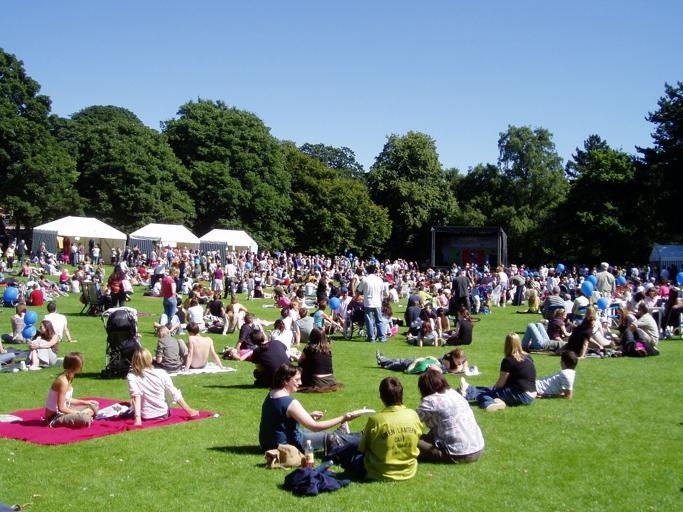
[122,279,134,295]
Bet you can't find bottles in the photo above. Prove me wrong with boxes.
[314,460,334,472]
[305,439,314,467]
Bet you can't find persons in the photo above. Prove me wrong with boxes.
[0,236,683,483]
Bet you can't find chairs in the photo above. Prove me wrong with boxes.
[79,276,106,316]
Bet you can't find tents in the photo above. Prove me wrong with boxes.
[32,215,259,264]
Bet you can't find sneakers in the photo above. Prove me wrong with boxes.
[459,377,469,397]
[486,400,506,412]
